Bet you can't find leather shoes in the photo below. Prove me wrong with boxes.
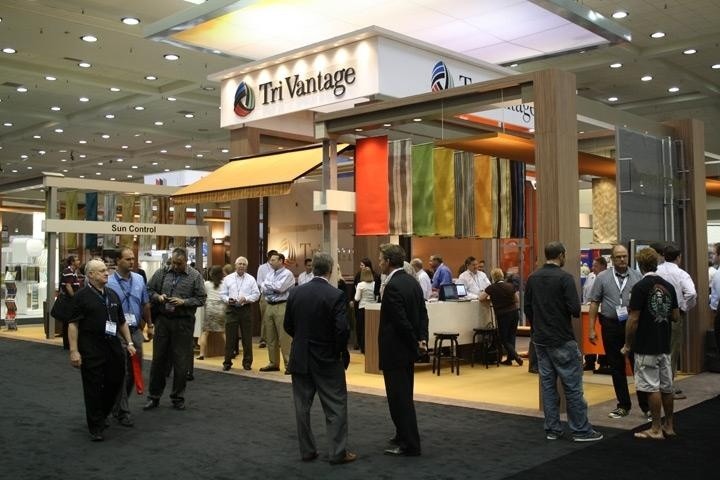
[88,429,104,442]
[142,397,161,411]
[384,445,422,459]
[117,415,136,426]
[222,361,292,376]
[172,400,187,411]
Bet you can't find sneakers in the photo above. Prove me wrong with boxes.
[572,430,605,443]
[302,450,320,463]
[330,452,359,463]
[545,427,563,441]
[608,407,630,419]
[391,434,402,445]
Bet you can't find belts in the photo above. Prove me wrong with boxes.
[266,299,287,306]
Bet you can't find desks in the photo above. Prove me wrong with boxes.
[365,297,496,375]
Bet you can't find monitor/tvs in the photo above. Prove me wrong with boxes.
[455,284,467,297]
[439,283,458,301]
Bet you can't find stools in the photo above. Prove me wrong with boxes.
[471,328,500,369]
[433,332,460,376]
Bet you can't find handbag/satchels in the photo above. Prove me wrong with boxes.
[50,293,72,322]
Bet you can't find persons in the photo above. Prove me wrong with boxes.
[427,254,523,366]
[60,245,295,441]
[283,243,430,465]
[523,241,719,441]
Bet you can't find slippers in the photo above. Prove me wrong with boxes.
[633,410,676,442]
